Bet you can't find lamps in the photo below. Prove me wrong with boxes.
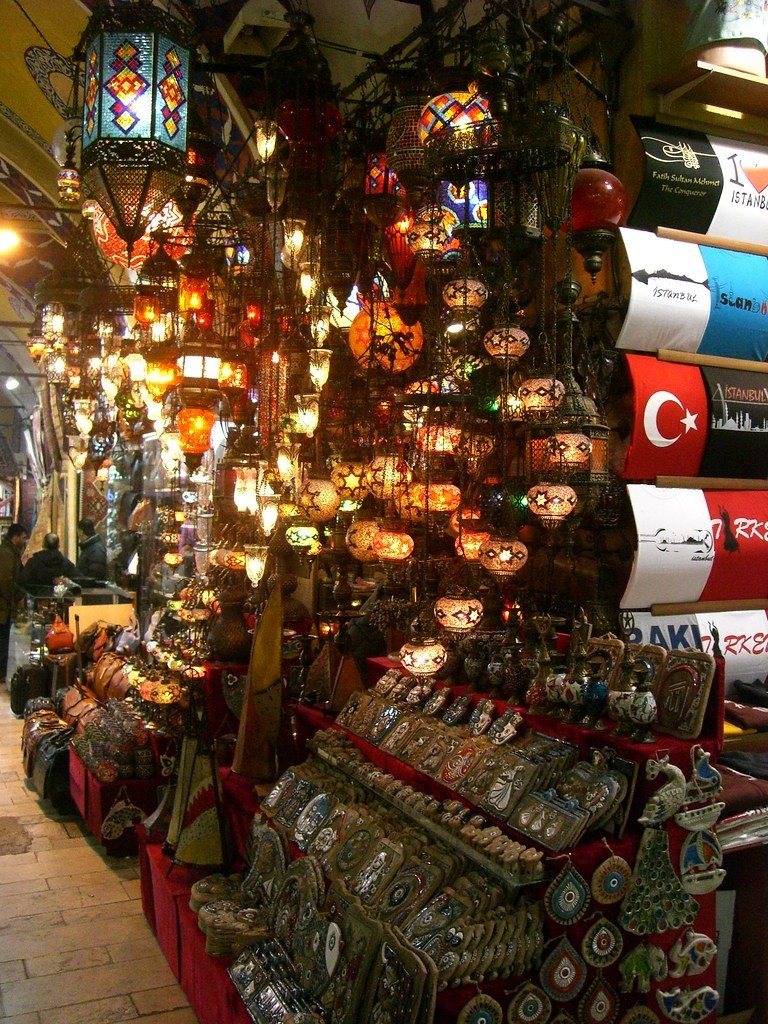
[0,0,598,727]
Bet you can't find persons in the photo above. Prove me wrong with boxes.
[0,524,27,693]
[15,532,75,613]
[76,519,109,588]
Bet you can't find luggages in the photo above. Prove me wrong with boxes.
[10,661,45,714]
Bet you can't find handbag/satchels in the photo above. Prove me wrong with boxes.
[21,709,76,798]
[62,621,142,734]
[44,614,73,653]
